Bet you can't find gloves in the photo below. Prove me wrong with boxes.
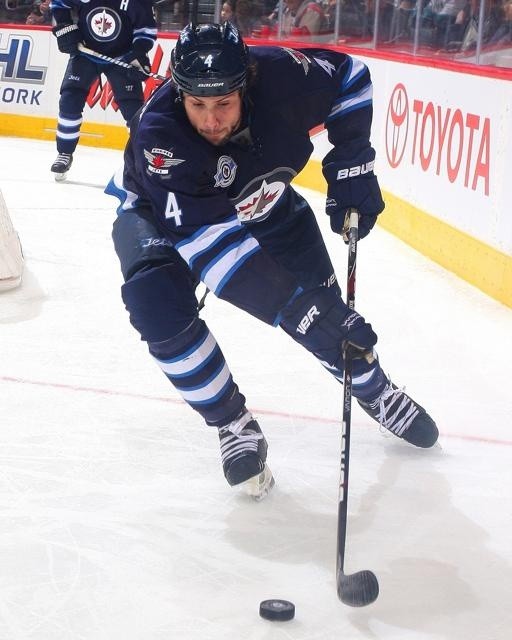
[280,289,377,373]
[120,40,153,81]
[321,149,385,243]
[52,21,84,55]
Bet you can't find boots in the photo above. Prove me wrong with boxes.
[204,395,267,484]
[366,383,439,448]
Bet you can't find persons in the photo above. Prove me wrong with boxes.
[50,0,158,173]
[113,23,438,485]
[1,0,512,54]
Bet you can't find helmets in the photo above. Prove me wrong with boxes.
[169,23,249,100]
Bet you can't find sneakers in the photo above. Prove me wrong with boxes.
[51,153,73,174]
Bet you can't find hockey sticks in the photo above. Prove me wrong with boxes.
[335,210,378,607]
